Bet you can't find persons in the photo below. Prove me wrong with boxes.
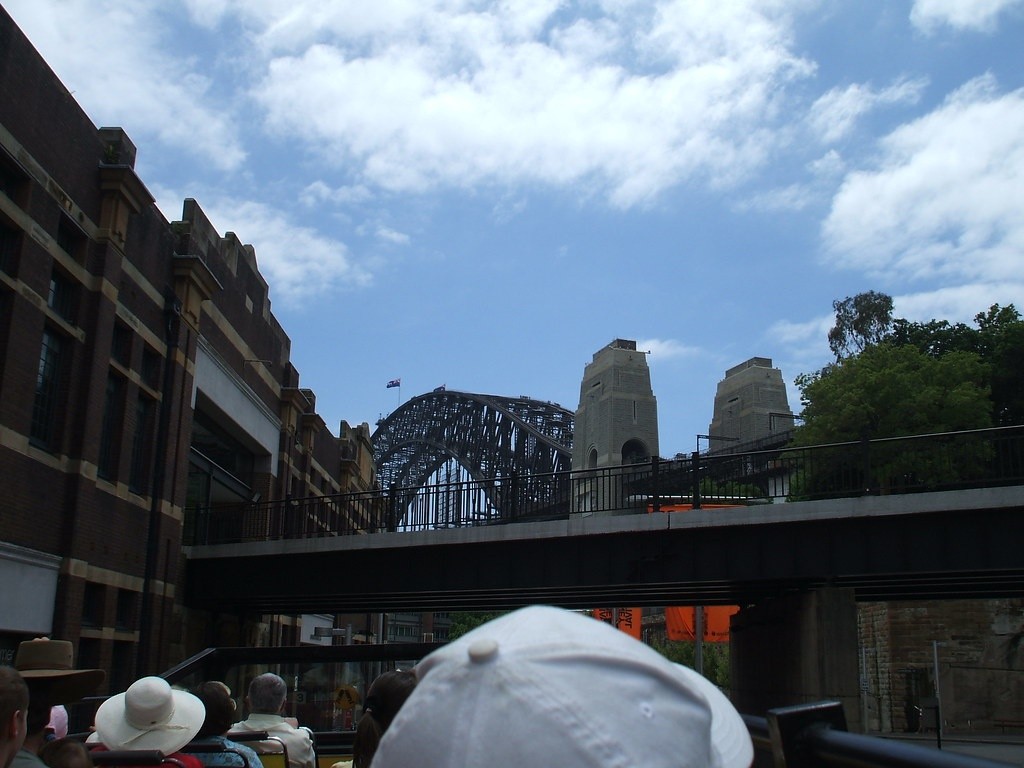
[0,636,420,768]
[368,603,755,768]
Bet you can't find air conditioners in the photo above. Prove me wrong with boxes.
[422,633,434,642]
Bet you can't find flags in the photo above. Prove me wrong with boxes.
[387,378,401,388]
[434,385,446,391]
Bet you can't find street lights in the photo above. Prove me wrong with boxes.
[924,639,948,749]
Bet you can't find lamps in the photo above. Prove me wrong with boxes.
[167,221,190,236]
[243,359,272,370]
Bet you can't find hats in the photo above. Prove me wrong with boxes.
[372,604,754,767]
[10,640,106,692]
[94,676,207,755]
